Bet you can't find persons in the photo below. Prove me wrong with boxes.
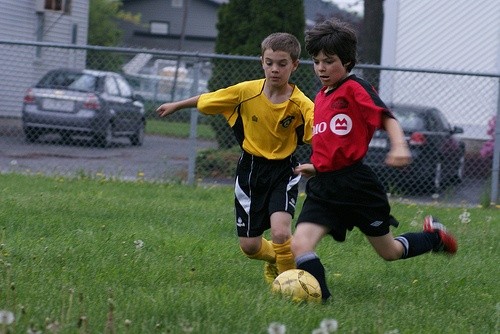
[156,32,315,285]
[290,16,458,305]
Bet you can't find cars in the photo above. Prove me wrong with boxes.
[361,102,468,196]
[124,47,214,108]
[22,67,147,149]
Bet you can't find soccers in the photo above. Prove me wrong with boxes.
[272,269,322,303]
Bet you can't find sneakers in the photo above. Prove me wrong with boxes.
[423,215,457,255]
[264,241,279,283]
